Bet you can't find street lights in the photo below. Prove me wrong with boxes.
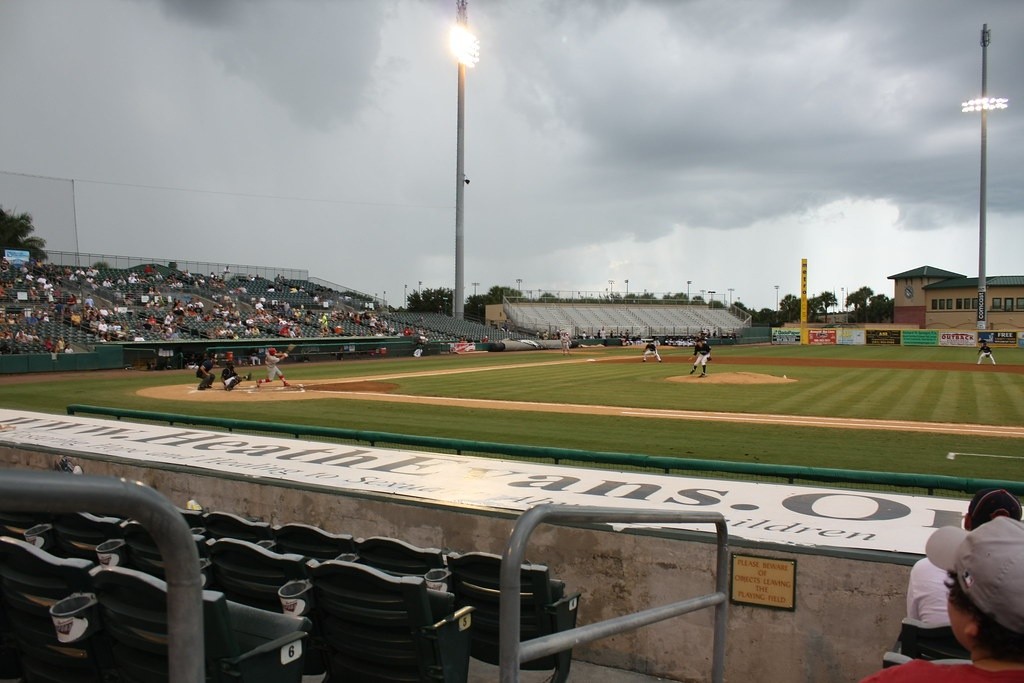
[471,283,480,296]
[702,289,715,309]
[688,280,692,300]
[961,97,1010,327]
[516,280,523,291]
[374,280,422,309]
[775,285,781,311]
[841,289,845,313]
[445,23,481,314]
[729,289,735,304]
[609,280,614,296]
[625,279,628,295]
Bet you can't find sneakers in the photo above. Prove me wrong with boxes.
[256,378,262,388]
[284,381,291,386]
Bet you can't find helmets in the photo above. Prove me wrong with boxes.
[269,348,276,355]
[226,360,235,365]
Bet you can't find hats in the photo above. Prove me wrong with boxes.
[967,488,1022,531]
[924,515,1024,633]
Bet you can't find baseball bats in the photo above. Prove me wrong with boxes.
[286,344,295,355]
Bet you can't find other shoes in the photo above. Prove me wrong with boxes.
[204,385,212,388]
[690,369,696,374]
[698,372,706,377]
[197,386,205,390]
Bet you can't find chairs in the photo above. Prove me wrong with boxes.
[0,265,743,354]
[0,504,583,683]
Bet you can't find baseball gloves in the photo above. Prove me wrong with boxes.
[243,372,252,381]
[707,357,712,361]
[986,354,990,357]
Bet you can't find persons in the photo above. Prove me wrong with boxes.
[977,339,996,366]
[458,334,497,343]
[642,342,661,362]
[196,353,218,390]
[494,320,512,334]
[857,487,1024,683]
[0,257,429,371]
[665,329,733,347]
[536,330,572,356]
[256,348,291,387]
[574,329,660,346]
[221,360,242,391]
[689,341,711,377]
